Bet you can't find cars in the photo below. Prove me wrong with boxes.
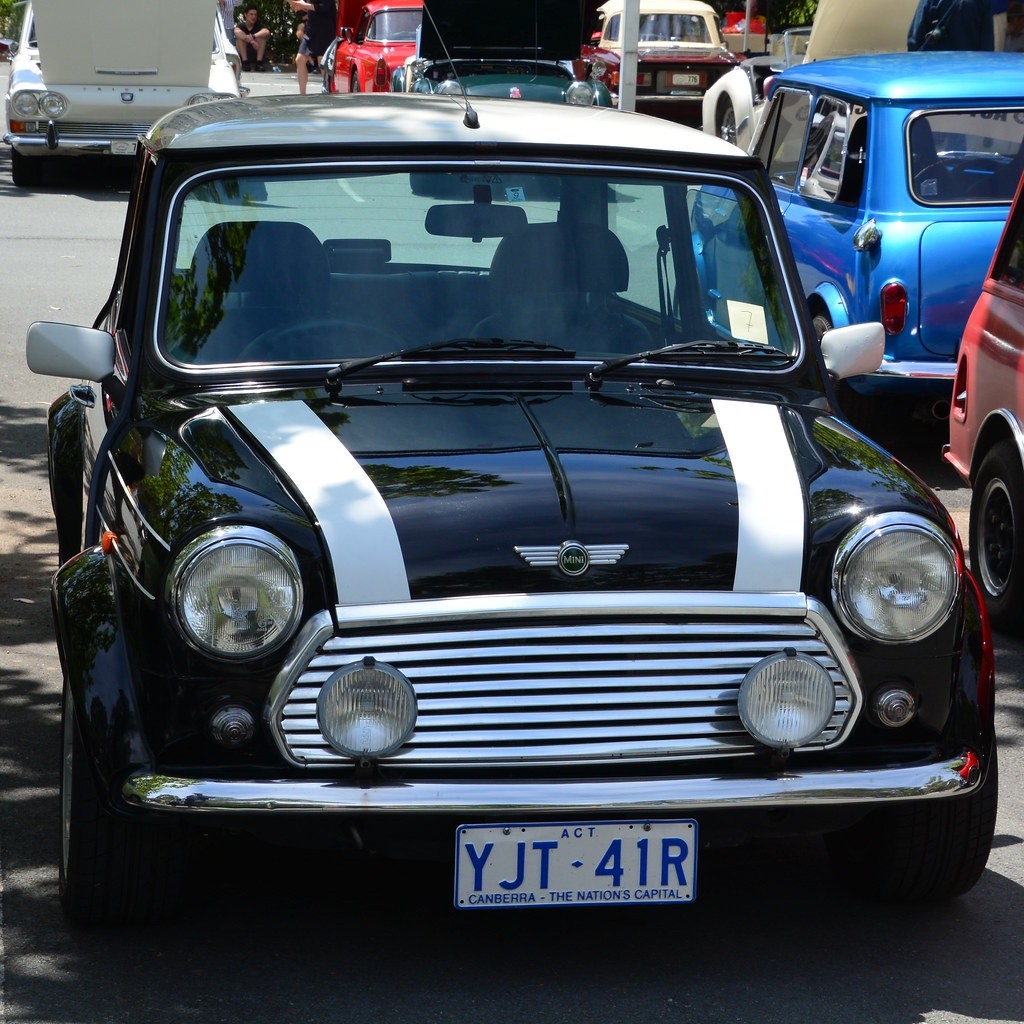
[938,172,1024,628]
[25,92,999,925]
[318,0,918,188]
[690,50,1024,432]
[0,1,250,189]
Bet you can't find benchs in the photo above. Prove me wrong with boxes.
[321,267,497,363]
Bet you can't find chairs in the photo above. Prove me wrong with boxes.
[832,116,955,210]
[179,221,345,362]
[471,221,655,354]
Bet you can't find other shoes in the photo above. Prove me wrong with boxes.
[243,62,250,71]
[255,64,265,71]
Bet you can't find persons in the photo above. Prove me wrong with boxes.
[217,0,242,46]
[290,0,336,95]
[234,4,270,72]
[722,0,771,34]
